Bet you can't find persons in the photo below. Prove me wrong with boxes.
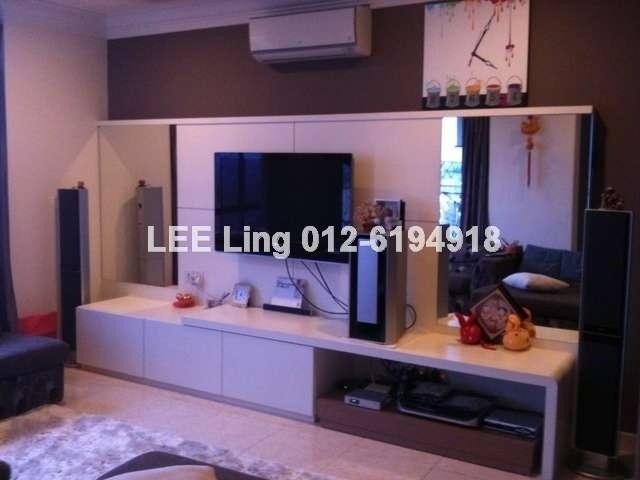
[480,304,508,333]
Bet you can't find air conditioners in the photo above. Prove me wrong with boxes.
[247,3,375,65]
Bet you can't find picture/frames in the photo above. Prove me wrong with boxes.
[468,285,529,345]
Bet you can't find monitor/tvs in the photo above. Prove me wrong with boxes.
[214,152,353,264]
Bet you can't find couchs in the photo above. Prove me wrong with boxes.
[471,245,581,329]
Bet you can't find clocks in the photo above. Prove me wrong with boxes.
[232,282,253,308]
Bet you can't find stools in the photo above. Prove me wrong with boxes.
[1,329,69,421]
[97,450,265,479]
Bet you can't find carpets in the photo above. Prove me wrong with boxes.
[1,402,325,480]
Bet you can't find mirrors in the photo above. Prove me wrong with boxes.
[436,113,597,343]
[96,125,173,288]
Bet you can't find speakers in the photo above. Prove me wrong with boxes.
[137,186,165,286]
[58,188,90,364]
[350,233,407,344]
[574,208,633,457]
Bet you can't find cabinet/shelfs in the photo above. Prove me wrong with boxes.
[75,297,580,480]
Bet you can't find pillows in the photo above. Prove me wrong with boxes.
[500,269,571,293]
[107,465,217,480]
